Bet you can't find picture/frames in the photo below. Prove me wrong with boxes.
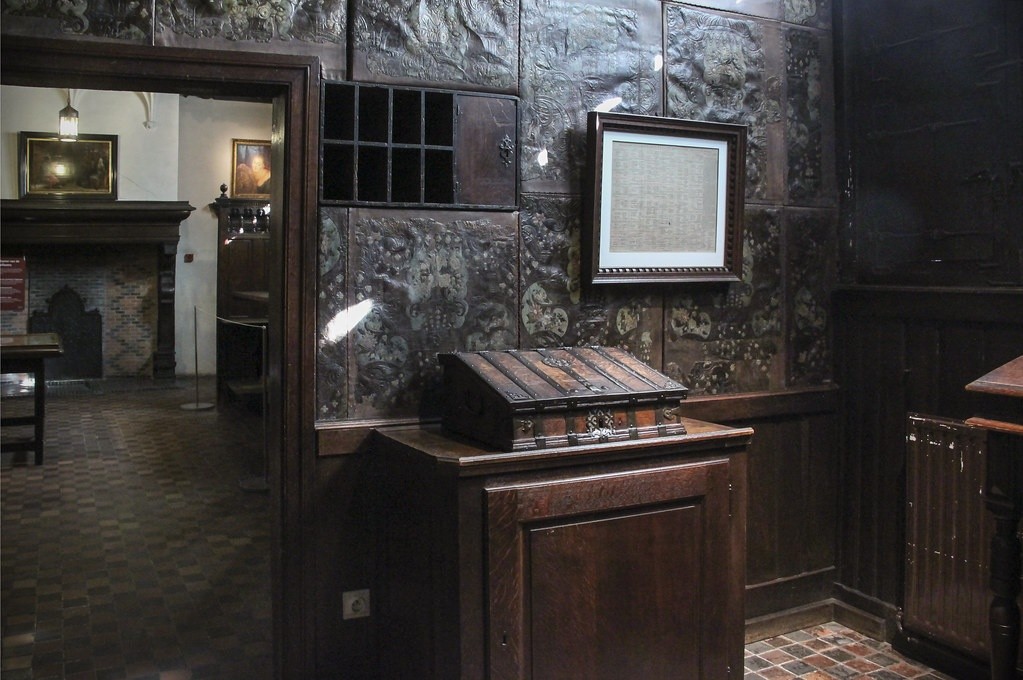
[582,111,749,284]
[17,131,119,199]
[230,138,272,199]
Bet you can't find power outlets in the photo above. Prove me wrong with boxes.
[342,588,370,620]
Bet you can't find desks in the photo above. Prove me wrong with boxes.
[964,354,1023,680]
[0,332,64,465]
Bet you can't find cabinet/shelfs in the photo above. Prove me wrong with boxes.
[371,413,753,680]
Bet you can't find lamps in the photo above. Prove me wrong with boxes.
[57,87,80,142]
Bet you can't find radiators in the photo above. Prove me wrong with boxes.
[899,411,994,664]
